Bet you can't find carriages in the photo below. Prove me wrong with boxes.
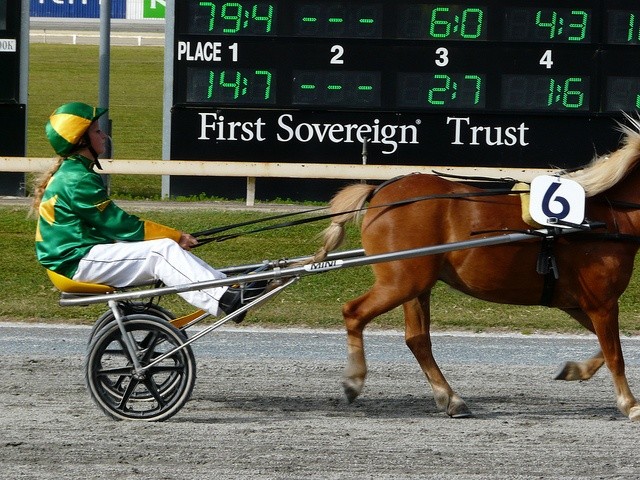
[60,108,640,422]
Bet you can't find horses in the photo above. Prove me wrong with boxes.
[282,109,639,421]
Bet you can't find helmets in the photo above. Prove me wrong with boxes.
[44,101,108,157]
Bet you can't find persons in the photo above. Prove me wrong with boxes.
[19,102,270,324]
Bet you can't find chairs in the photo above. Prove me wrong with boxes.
[45,268,116,295]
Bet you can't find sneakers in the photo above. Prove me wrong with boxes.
[227,268,271,288]
[218,280,267,324]
